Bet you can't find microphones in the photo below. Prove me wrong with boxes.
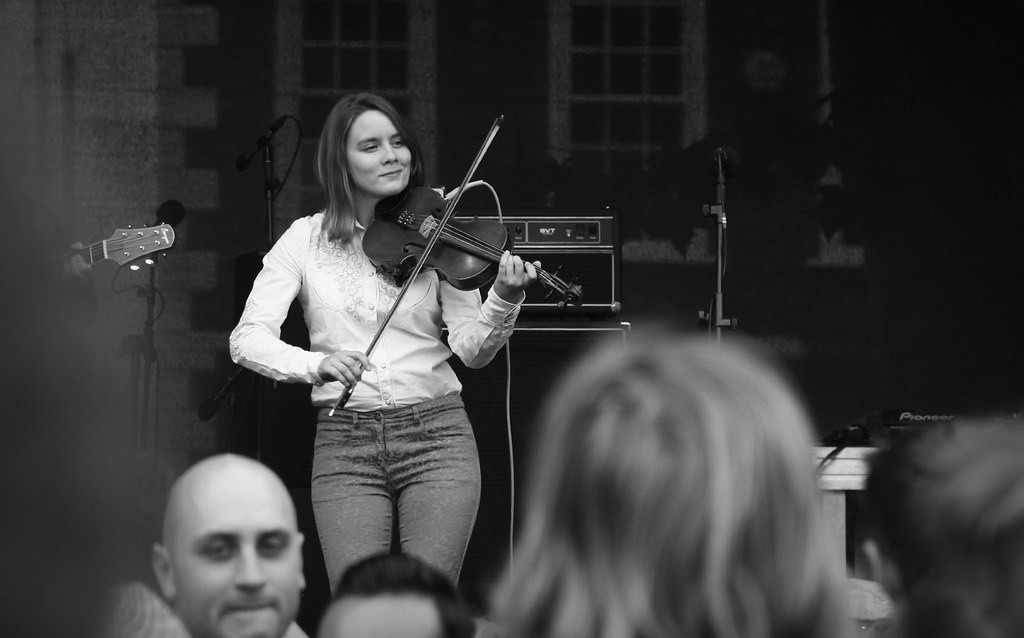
[153,198,187,231]
[234,115,289,172]
[196,366,245,422]
[707,146,740,179]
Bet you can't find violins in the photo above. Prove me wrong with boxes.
[360,183,585,310]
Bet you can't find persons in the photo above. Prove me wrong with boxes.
[230,90,544,600]
[148,452,310,638]
[317,550,478,637]
[861,414,1022,638]
[477,324,862,638]
